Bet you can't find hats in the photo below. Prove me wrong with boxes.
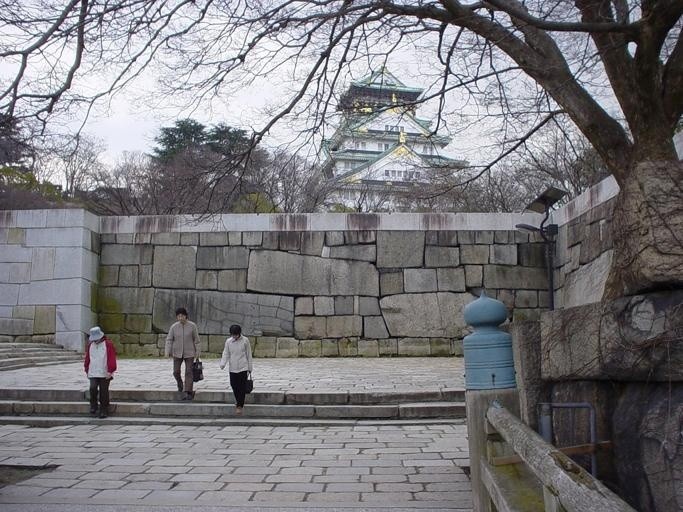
[89,326,104,341]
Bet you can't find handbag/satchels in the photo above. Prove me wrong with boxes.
[193,358,203,382]
[245,373,253,394]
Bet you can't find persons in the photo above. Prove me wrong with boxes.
[220,325,253,416]
[84,327,116,418]
[165,307,201,400]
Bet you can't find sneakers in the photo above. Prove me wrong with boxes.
[236,406,242,415]
[182,391,192,400]
[90,406,107,417]
[178,382,183,392]
